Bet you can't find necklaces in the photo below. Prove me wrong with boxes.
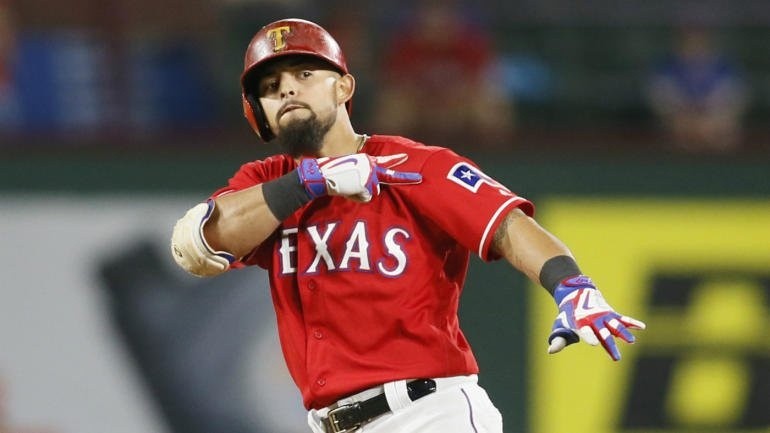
[356,133,367,153]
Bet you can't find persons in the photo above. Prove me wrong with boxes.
[170,17,649,433]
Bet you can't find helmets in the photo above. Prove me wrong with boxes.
[241,18,354,143]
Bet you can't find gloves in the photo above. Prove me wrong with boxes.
[296,152,422,203]
[546,275,645,364]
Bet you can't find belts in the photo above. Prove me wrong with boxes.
[321,377,437,433]
